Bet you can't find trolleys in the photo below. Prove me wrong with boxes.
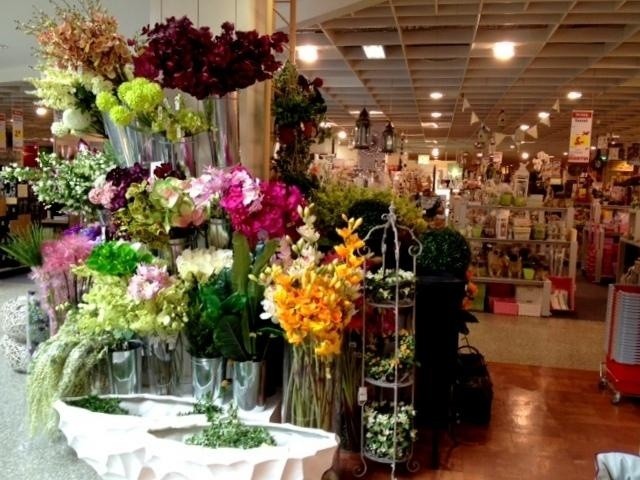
[596,264,640,408]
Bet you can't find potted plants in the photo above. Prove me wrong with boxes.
[51,387,342,480]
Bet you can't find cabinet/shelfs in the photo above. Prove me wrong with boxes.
[425,154,640,316]
